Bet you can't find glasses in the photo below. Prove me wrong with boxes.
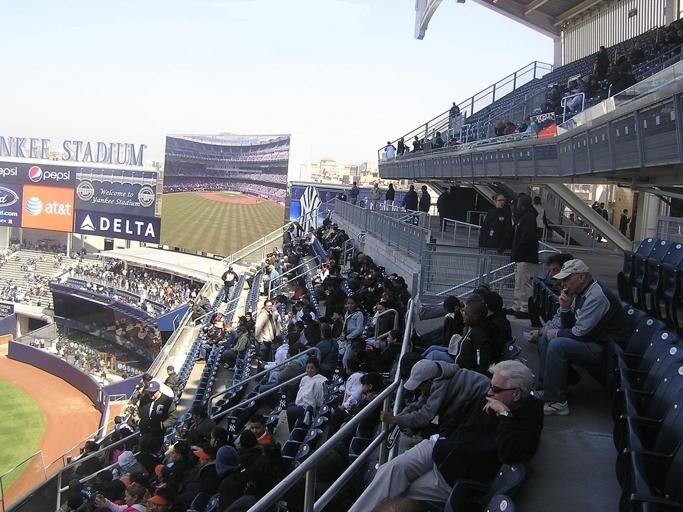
[489,382,523,393]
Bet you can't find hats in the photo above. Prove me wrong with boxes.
[145,381,160,392]
[359,371,381,385]
[146,482,177,506]
[155,464,172,481]
[216,446,240,475]
[403,358,439,391]
[552,259,590,280]
[193,447,215,460]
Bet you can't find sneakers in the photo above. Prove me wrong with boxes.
[505,308,519,315]
[544,401,570,417]
[514,311,529,319]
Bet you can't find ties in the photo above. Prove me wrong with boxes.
[269,314,276,345]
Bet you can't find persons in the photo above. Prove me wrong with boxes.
[349,182,359,204]
[450,137,460,146]
[369,182,384,211]
[433,132,443,148]
[58,437,111,511]
[400,185,418,213]
[396,137,409,155]
[412,136,421,150]
[493,28,683,136]
[384,141,396,161]
[450,102,461,119]
[0,234,110,382]
[163,134,290,202]
[419,185,431,213]
[437,186,452,232]
[111,191,637,512]
[385,183,396,211]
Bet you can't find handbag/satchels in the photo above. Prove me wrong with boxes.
[479,217,514,250]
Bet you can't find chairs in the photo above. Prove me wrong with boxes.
[451,18,683,144]
